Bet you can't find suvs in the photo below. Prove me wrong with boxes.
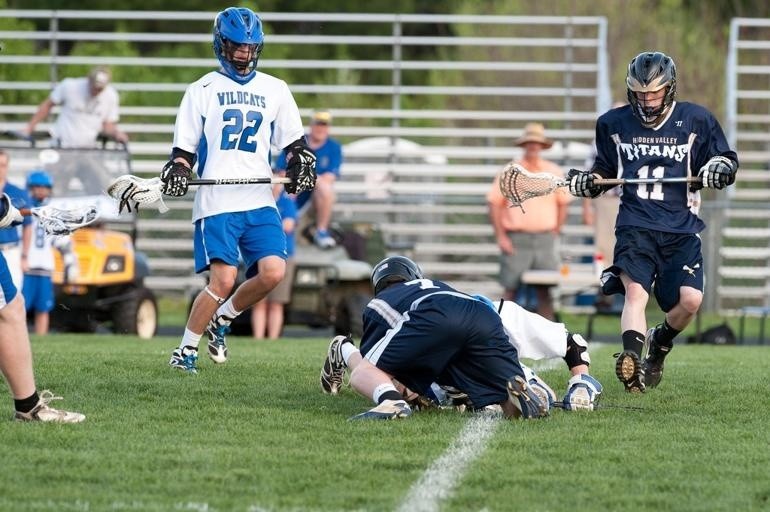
[1,136,160,338]
[187,202,372,334]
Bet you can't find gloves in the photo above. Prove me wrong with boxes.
[161,160,193,195]
[695,156,738,190]
[284,144,316,195]
[565,167,600,197]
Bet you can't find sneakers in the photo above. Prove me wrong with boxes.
[204,311,235,365]
[14,390,85,424]
[307,225,336,250]
[168,346,202,377]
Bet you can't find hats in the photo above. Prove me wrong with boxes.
[312,111,331,125]
[513,122,554,149]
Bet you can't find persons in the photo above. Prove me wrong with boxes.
[565,51,739,396]
[274,112,343,250]
[0,151,34,294]
[20,67,128,193]
[320,292,603,415]
[161,6,316,376]
[0,191,87,426]
[348,252,551,429]
[580,98,629,273]
[484,141,574,322]
[250,168,299,340]
[21,171,78,336]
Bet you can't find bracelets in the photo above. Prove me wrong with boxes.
[22,254,28,259]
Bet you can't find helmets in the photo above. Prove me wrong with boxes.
[626,52,677,129]
[213,7,264,84]
[27,172,52,188]
